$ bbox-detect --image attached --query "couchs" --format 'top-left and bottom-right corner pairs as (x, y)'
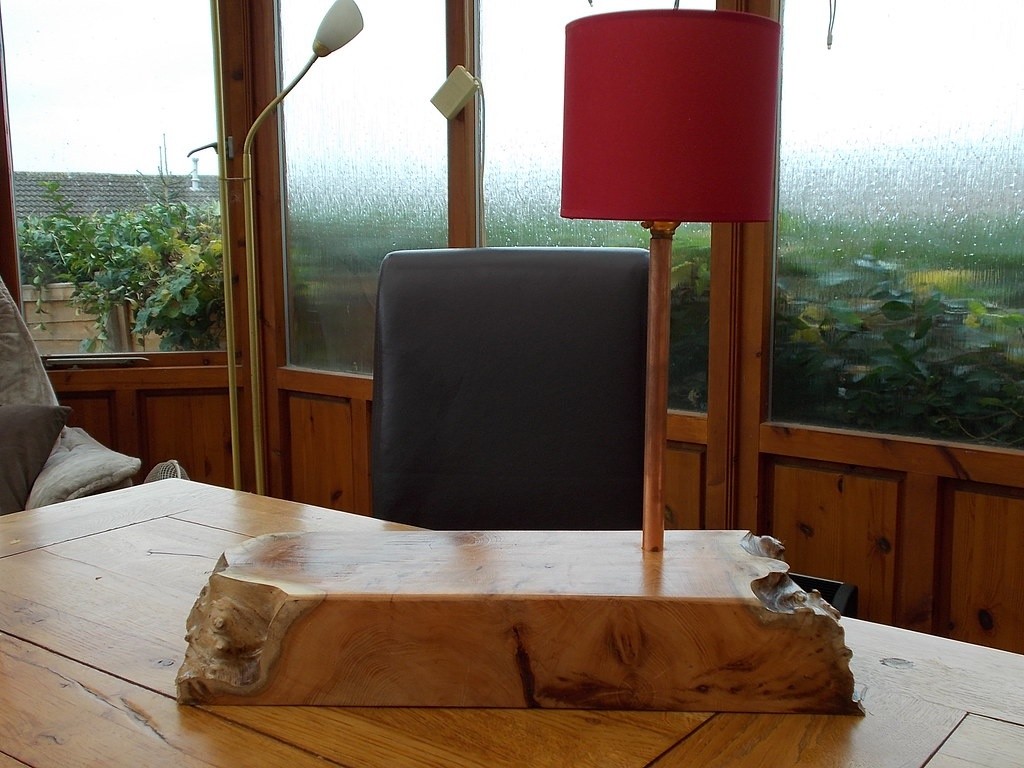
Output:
(0, 276), (142, 511)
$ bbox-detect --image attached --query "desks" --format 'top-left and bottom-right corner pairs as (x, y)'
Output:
(0, 475), (1024, 768)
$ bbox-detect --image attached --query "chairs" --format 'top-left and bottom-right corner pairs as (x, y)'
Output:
(367, 246), (647, 532)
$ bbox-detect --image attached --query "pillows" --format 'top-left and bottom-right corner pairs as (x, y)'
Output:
(0, 402), (73, 516)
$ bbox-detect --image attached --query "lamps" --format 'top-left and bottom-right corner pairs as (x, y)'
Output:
(174, 10), (866, 706)
(242, 0), (364, 497)
